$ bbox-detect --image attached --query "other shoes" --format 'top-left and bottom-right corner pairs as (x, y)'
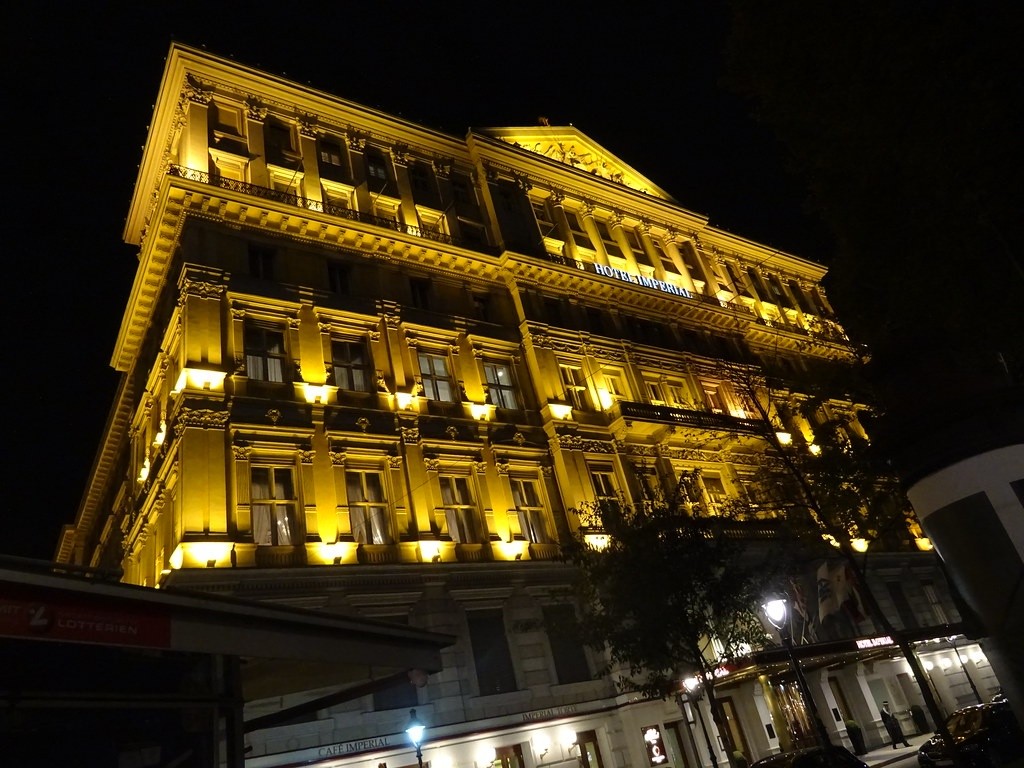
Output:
(906, 745), (913, 747)
(894, 747), (897, 749)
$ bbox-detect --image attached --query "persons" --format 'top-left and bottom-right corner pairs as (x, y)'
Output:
(795, 724), (804, 739)
(732, 751), (749, 768)
(880, 701), (913, 749)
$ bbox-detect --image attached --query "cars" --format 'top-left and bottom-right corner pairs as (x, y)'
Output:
(917, 690), (1012, 768)
(750, 744), (869, 768)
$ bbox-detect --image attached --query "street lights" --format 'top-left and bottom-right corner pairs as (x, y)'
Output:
(405, 707), (426, 768)
(761, 592), (834, 744)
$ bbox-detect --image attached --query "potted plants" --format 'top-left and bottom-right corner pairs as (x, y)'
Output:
(732, 750), (748, 768)
(846, 718), (867, 757)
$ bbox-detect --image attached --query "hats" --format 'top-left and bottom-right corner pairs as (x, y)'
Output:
(882, 701), (888, 705)
